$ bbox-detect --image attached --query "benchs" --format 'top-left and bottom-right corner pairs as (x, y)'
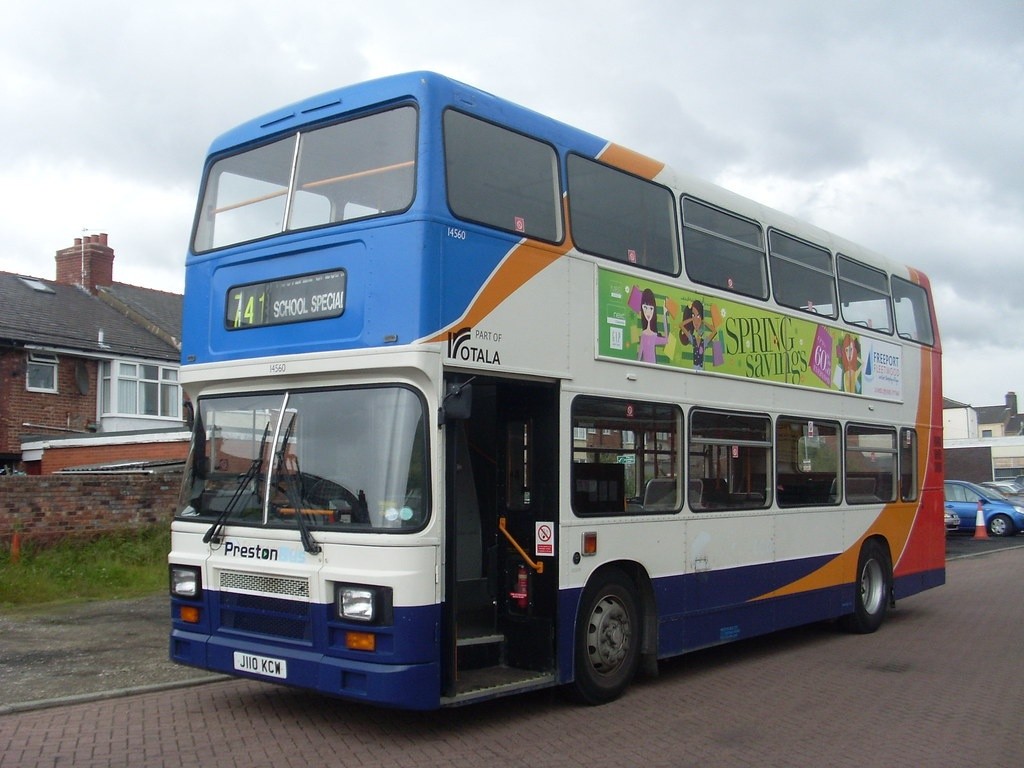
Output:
(625, 471), (913, 510)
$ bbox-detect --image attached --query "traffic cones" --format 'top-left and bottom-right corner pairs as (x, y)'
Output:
(8, 529), (22, 562)
(969, 499), (994, 542)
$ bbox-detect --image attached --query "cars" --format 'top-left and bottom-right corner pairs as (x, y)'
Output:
(978, 475), (1024, 508)
(943, 478), (1024, 538)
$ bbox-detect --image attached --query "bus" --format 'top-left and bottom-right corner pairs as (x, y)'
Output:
(165, 69), (948, 714)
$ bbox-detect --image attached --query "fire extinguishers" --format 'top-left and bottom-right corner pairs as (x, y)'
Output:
(518, 564), (528, 608)
